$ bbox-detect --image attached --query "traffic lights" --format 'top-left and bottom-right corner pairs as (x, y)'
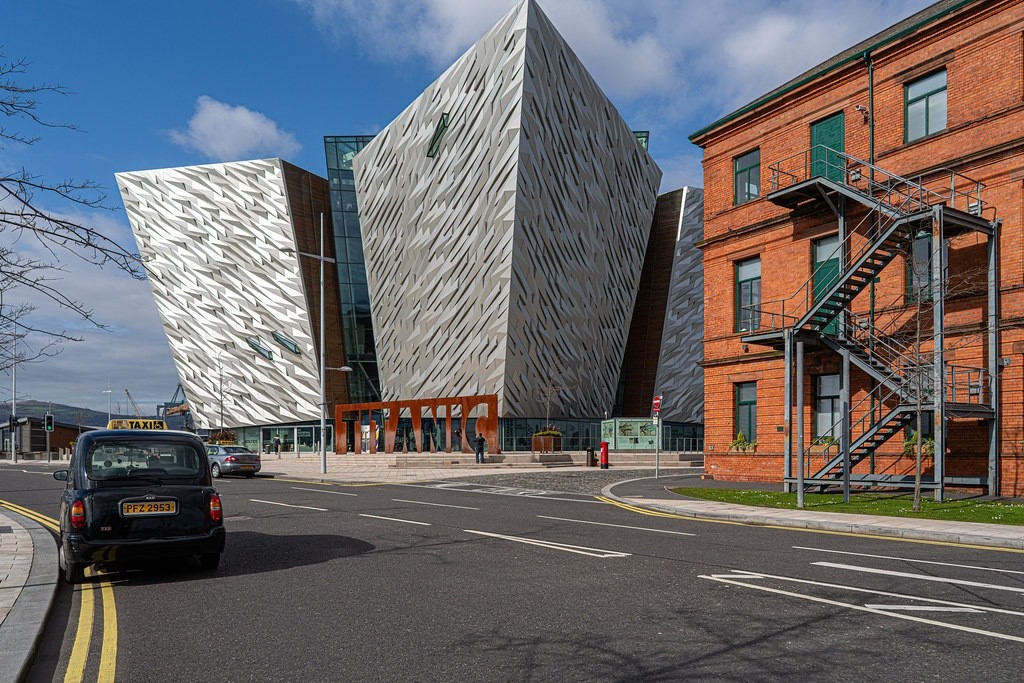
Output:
(44, 414), (54, 432)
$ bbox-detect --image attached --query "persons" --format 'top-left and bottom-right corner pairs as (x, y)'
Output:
(273, 434), (281, 455)
(473, 433), (486, 463)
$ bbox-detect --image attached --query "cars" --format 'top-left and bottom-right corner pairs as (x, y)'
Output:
(52, 419), (225, 585)
(205, 443), (262, 479)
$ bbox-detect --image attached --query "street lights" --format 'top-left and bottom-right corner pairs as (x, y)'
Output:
(280, 212), (353, 473)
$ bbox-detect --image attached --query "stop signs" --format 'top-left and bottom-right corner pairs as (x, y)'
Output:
(653, 396), (660, 407)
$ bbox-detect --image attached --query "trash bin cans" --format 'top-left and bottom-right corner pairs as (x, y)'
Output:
(265, 445), (271, 454)
(586, 446), (594, 467)
